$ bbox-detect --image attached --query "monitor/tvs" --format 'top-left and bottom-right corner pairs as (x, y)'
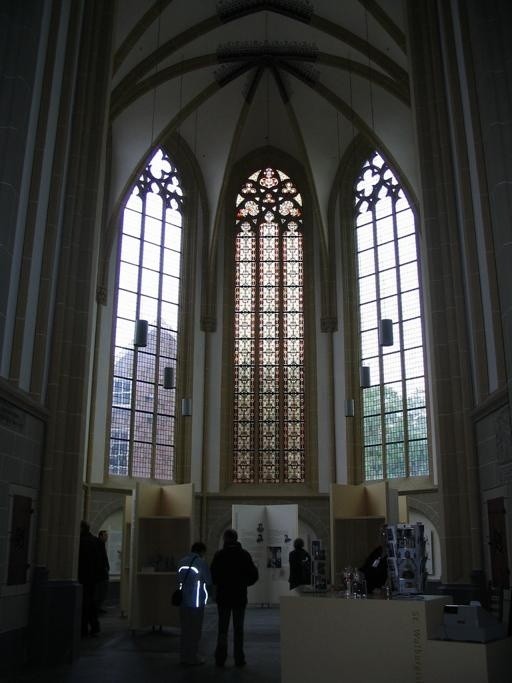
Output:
(433, 583), (488, 609)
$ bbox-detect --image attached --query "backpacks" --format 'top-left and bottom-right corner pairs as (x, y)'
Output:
(171, 589), (183, 607)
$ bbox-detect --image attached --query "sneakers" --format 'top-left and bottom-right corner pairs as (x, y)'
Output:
(179, 655), (207, 665)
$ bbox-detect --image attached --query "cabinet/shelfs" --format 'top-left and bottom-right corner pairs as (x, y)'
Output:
(329, 482), (389, 586)
(127, 481), (195, 636)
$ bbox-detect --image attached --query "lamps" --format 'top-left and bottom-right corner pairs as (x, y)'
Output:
(181, 399), (192, 416)
(345, 399), (354, 417)
(134, 320), (148, 347)
(164, 367), (174, 389)
(378, 319), (393, 346)
(360, 367), (370, 388)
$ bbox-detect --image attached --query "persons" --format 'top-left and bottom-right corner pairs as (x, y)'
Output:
(288, 538), (311, 590)
(177, 542), (212, 666)
(210, 529), (259, 667)
(95, 530), (110, 614)
(343, 541), (389, 595)
(80, 521), (107, 639)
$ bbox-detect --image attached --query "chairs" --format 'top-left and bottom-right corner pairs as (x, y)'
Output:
(485, 580), (504, 620)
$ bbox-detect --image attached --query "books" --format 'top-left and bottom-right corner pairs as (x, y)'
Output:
(383, 516), (430, 593)
